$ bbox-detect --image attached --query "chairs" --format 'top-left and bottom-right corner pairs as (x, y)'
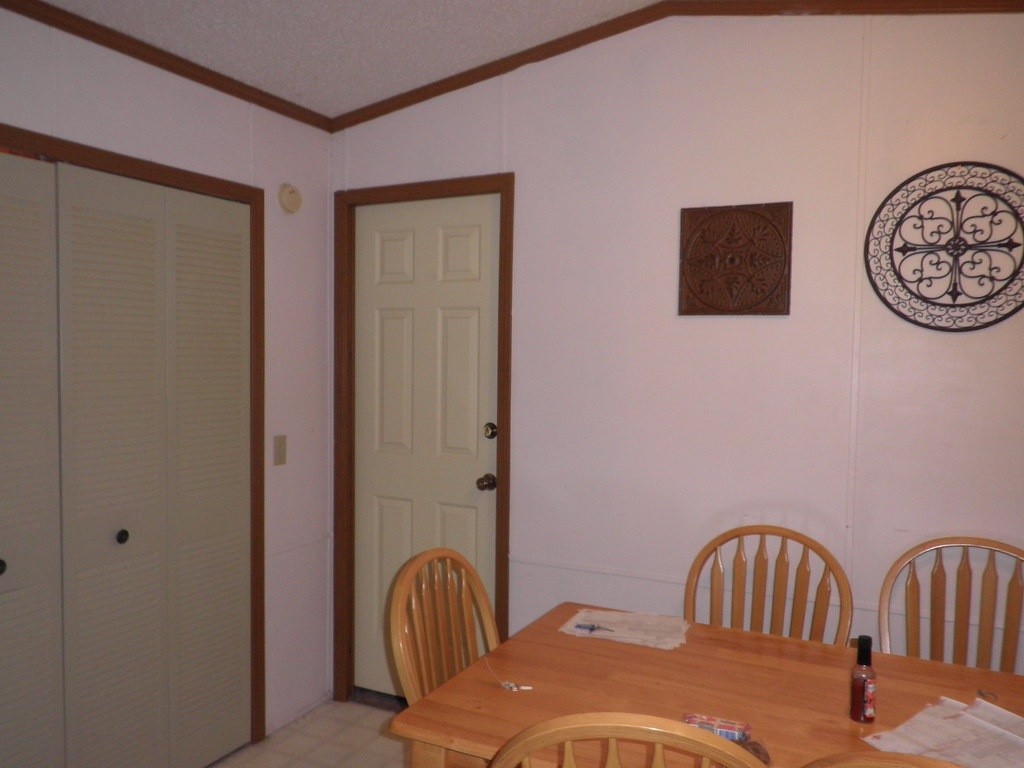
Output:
(878, 537), (1024, 674)
(488, 712), (767, 768)
(381, 548), (502, 709)
(684, 525), (852, 648)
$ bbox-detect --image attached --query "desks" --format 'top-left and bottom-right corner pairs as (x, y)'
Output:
(389, 602), (1024, 768)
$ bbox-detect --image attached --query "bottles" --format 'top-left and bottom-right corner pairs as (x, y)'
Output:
(850, 636), (878, 723)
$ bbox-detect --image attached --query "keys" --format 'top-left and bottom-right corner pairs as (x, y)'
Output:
(501, 681), (534, 692)
(575, 624), (615, 634)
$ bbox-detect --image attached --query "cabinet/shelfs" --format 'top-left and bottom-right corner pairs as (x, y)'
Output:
(0, 151), (251, 767)
